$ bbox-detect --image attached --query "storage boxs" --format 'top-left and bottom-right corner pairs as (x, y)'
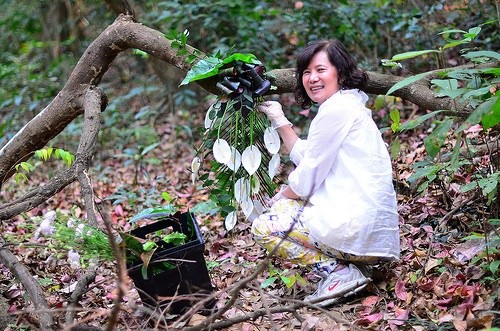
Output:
(125, 211), (215, 328)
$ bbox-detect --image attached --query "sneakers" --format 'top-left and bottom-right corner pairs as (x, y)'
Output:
(302, 263), (372, 309)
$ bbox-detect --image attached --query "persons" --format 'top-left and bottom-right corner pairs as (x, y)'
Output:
(251, 37), (400, 308)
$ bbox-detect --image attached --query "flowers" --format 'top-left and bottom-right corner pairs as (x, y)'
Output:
(178, 52), (284, 232)
